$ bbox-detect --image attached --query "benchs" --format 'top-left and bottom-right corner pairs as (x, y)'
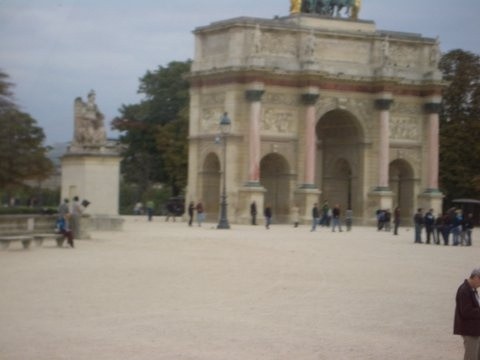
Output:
(34, 233), (63, 248)
(0, 236), (33, 250)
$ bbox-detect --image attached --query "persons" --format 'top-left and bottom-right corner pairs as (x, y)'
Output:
(310, 199), (353, 233)
(452, 268), (480, 360)
(250, 201), (258, 225)
(55, 195), (91, 247)
(133, 198), (144, 219)
(414, 207), (474, 246)
(291, 203), (300, 228)
(376, 205), (401, 235)
(263, 202), (272, 229)
(164, 200), (175, 222)
(187, 200), (204, 227)
(147, 200), (155, 220)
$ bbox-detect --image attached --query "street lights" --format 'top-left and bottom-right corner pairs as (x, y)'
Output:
(215, 112), (231, 228)
